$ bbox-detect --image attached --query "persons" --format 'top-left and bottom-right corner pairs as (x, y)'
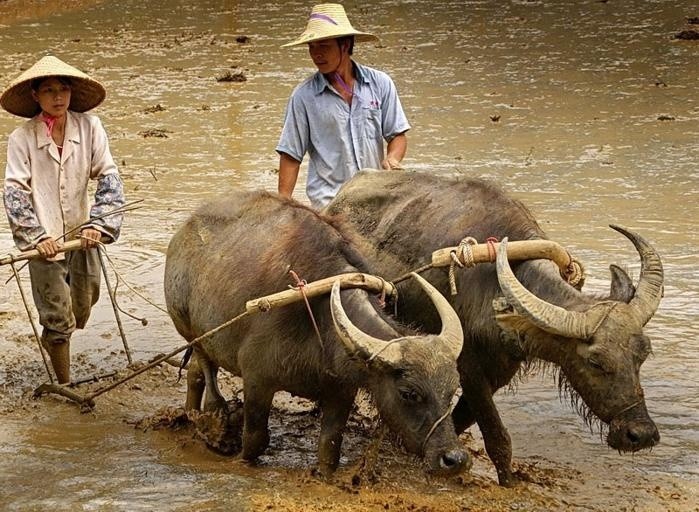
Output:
(275, 3), (412, 215)
(0, 54), (127, 386)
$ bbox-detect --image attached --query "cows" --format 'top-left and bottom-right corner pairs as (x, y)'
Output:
(316, 167), (665, 490)
(163, 189), (474, 481)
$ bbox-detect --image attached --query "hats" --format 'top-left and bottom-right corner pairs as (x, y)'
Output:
(0, 56), (106, 118)
(279, 3), (379, 50)
